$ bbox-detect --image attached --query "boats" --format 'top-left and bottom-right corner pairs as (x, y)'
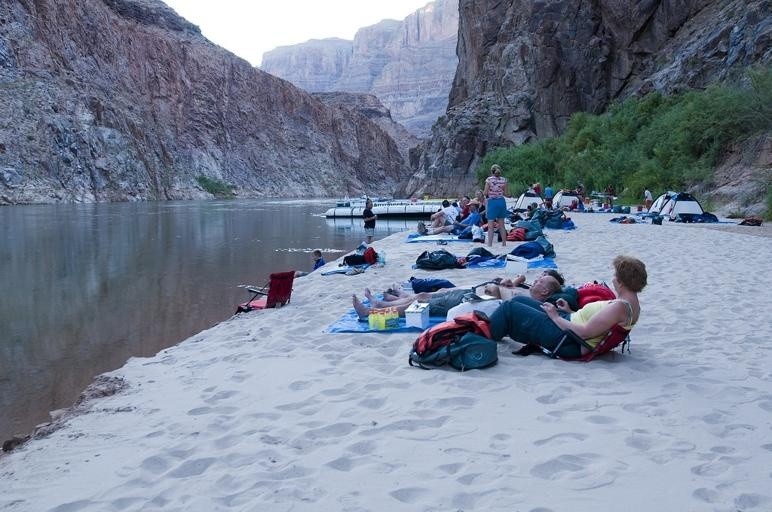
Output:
(325, 200), (454, 217)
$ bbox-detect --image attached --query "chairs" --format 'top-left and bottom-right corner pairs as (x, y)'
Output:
(526, 323), (632, 361)
(235, 271), (295, 314)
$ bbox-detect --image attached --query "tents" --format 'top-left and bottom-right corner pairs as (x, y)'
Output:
(648, 190), (704, 221)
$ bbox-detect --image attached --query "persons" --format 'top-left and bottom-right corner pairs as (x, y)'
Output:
(604, 184), (614, 207)
(351, 275), (561, 320)
(417, 164), (585, 247)
(383, 268), (565, 301)
(643, 187), (653, 210)
(293, 249), (326, 278)
(487, 255), (647, 359)
(362, 199), (378, 244)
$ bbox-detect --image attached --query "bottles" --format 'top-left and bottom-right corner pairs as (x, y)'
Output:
(368, 306), (398, 329)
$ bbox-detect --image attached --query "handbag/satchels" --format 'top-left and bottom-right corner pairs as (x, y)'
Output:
(344, 241), (378, 264)
(458, 225), (483, 241)
(409, 310), (498, 372)
